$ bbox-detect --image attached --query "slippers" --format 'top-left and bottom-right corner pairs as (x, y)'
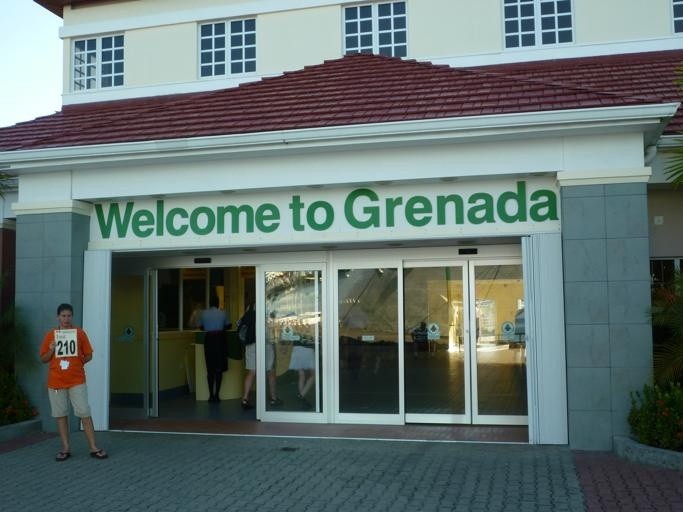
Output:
(91, 449), (107, 458)
(56, 452), (70, 461)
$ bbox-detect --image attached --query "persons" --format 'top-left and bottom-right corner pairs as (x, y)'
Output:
(289, 333), (315, 401)
(198, 296), (232, 404)
(187, 302), (201, 328)
(39, 303), (107, 461)
(236, 310), (284, 408)
(246, 296), (273, 340)
(411, 322), (428, 358)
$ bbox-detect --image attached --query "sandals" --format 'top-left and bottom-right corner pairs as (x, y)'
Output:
(270, 398), (283, 406)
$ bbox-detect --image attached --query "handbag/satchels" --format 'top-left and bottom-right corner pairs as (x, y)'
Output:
(236, 301), (255, 341)
